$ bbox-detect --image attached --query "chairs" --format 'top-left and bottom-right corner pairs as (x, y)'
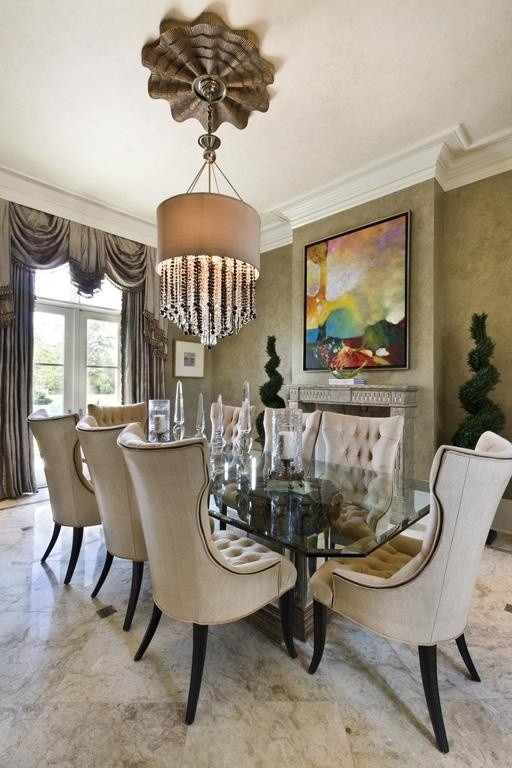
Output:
(306, 430), (512, 753)
(27, 410), (104, 584)
(88, 403), (150, 446)
(74, 415), (148, 634)
(209, 404), (406, 564)
(116, 422), (302, 726)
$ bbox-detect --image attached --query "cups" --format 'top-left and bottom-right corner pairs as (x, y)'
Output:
(149, 399), (170, 443)
(236, 457), (257, 493)
(272, 407), (304, 482)
(270, 492), (297, 542)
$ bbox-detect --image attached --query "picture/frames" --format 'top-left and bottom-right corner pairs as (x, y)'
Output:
(303, 211), (410, 372)
(174, 339), (206, 379)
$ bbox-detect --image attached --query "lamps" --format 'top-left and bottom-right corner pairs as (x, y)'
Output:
(142, 23), (273, 350)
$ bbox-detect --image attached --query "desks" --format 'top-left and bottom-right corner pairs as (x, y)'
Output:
(158, 439), (434, 646)
